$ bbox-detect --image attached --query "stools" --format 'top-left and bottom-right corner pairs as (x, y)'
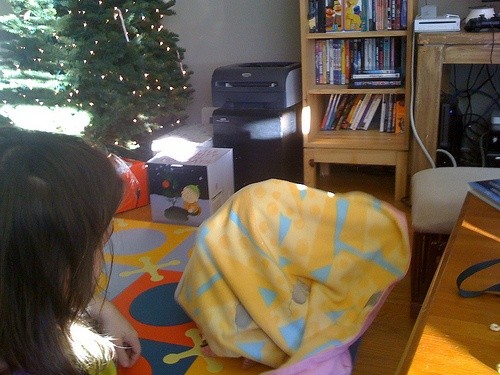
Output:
(411, 166), (499, 316)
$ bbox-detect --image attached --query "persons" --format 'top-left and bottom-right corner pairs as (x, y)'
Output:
(0, 127), (140, 375)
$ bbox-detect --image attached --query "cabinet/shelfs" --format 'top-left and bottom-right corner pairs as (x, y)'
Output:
(299, 0), (418, 200)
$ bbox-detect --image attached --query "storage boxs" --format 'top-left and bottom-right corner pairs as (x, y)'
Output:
(147, 148), (234, 226)
(113, 155), (150, 214)
(213, 103), (302, 192)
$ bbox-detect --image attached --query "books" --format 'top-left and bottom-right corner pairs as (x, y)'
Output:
(467, 178), (500, 211)
(308, 0), (407, 134)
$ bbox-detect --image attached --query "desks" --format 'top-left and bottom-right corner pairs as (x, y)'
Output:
(410, 32), (500, 176)
(395, 192), (500, 375)
